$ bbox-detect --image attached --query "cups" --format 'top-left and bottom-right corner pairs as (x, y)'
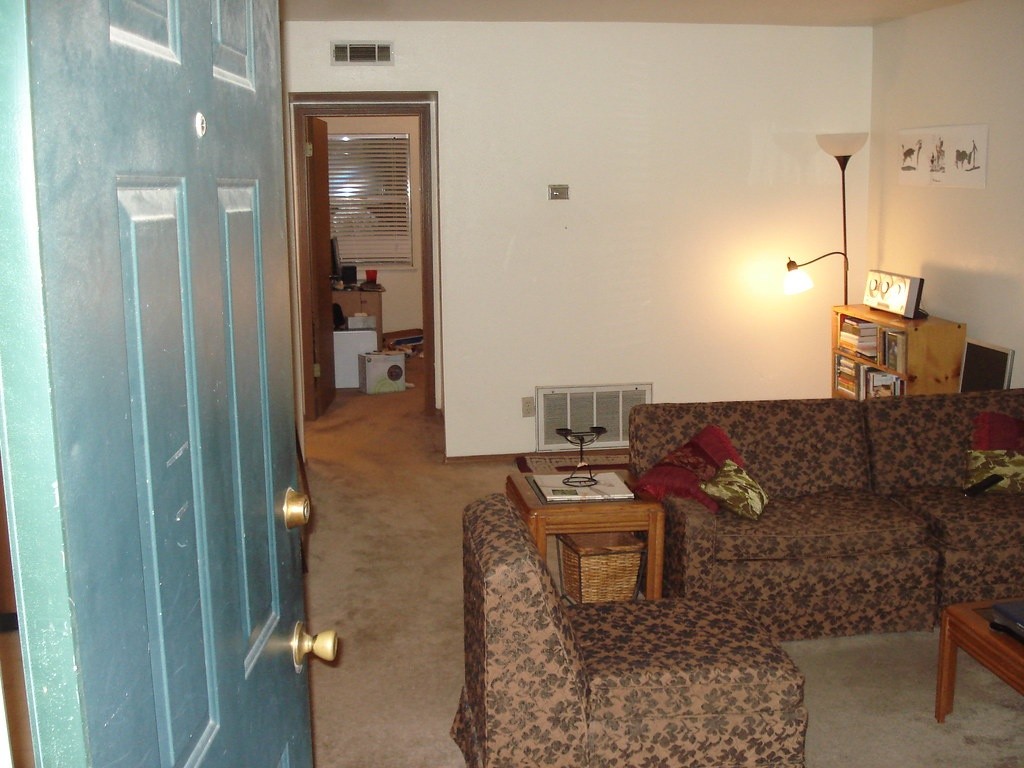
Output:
(365, 270), (377, 285)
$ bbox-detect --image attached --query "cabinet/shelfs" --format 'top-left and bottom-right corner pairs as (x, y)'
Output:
(332, 283), (386, 353)
(830, 304), (967, 401)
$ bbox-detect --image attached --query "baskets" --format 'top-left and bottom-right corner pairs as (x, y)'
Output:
(555, 531), (644, 604)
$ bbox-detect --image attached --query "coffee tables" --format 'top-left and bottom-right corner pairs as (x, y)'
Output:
(934, 597), (1024, 723)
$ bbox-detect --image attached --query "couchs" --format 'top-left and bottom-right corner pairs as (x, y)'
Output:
(628, 387), (1024, 643)
(451, 492), (809, 768)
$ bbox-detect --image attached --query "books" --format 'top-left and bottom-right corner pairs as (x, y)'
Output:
(837, 317), (905, 399)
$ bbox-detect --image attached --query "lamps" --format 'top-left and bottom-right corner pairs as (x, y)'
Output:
(782, 132), (870, 305)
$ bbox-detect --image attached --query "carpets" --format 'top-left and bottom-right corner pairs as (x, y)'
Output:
(516, 454), (630, 473)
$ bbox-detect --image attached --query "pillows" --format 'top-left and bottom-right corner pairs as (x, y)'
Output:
(698, 459), (770, 522)
(635, 422), (746, 514)
(971, 411), (1024, 452)
(962, 449), (1024, 494)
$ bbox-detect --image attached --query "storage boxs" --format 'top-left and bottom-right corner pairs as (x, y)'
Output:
(333, 331), (377, 389)
(357, 353), (406, 394)
(345, 316), (376, 330)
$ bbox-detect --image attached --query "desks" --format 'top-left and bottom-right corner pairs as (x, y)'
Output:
(502, 469), (667, 599)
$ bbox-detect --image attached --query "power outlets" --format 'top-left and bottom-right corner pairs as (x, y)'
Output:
(522, 397), (535, 417)
